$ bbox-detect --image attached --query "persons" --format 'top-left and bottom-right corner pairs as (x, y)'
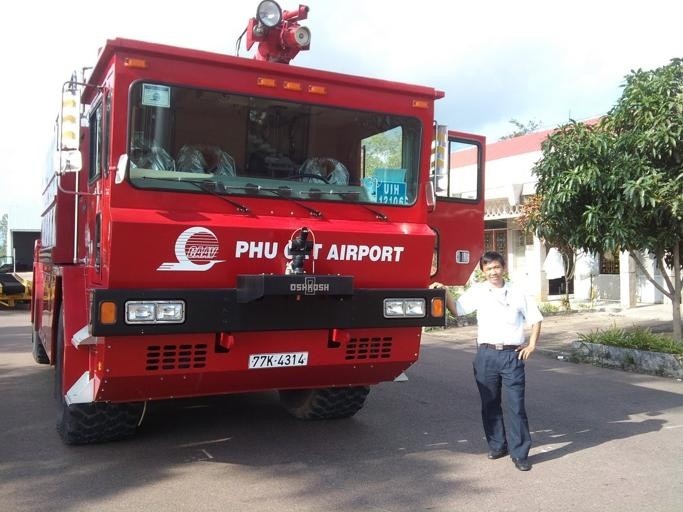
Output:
(427, 251), (544, 471)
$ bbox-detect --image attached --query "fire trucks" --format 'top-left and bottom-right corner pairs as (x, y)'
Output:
(31, 0), (486, 446)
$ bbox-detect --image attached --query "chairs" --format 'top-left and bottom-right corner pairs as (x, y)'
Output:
(141, 141), (348, 193)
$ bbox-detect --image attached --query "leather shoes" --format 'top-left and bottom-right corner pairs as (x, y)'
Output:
(512, 457), (531, 471)
(488, 447), (509, 460)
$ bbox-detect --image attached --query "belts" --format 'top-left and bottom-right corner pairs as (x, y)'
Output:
(479, 343), (522, 352)
